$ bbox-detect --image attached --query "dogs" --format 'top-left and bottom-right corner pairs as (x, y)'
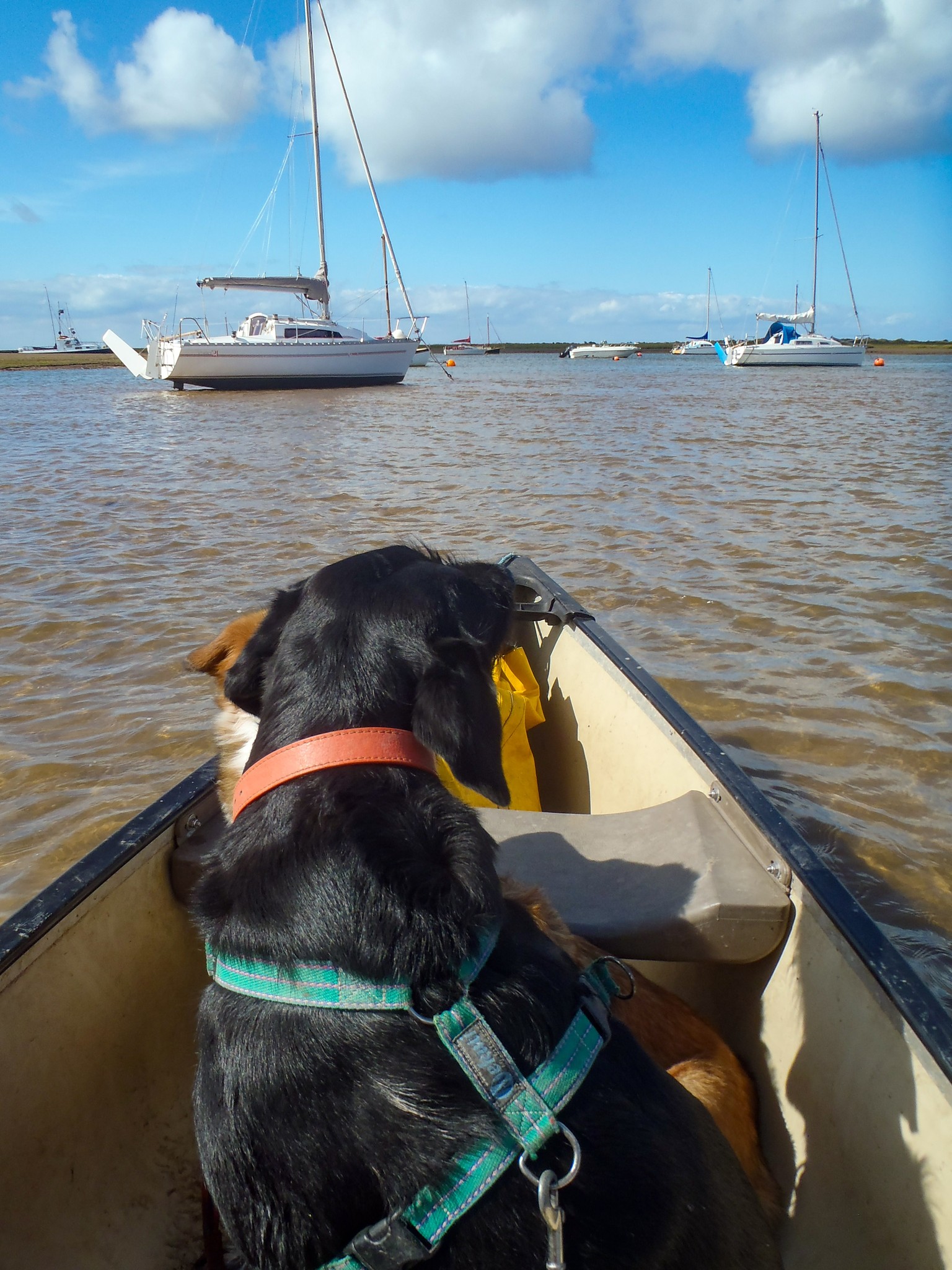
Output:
(165, 533), (791, 1270)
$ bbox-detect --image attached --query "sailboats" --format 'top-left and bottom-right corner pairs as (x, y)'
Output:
(18, 283), (114, 354)
(713, 108), (870, 368)
(669, 265), (719, 354)
(442, 280), (506, 356)
(101, 0), (430, 390)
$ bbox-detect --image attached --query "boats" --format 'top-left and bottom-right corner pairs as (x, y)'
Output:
(376, 233), (430, 371)
(558, 340), (643, 358)
